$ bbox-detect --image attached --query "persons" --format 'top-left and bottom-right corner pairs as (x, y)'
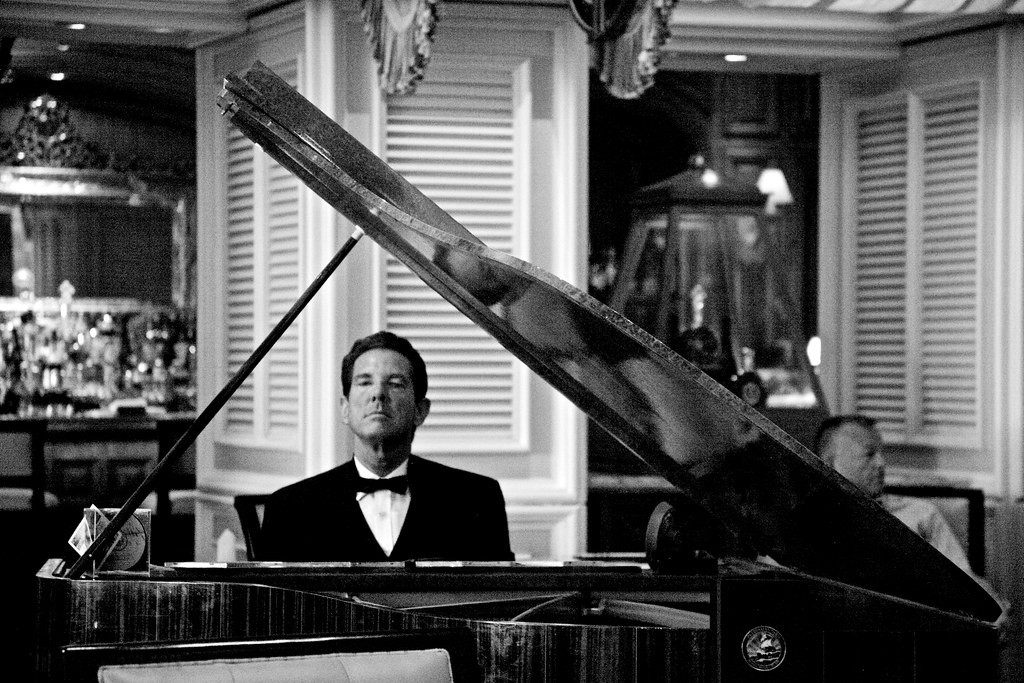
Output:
(815, 414), (975, 580)
(257, 330), (518, 562)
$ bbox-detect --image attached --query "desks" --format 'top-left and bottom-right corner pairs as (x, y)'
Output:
(0, 416), (197, 511)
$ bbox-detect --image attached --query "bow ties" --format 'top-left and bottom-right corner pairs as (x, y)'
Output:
(355, 474), (409, 495)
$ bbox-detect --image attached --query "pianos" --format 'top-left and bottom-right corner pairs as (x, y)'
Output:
(29, 59), (1014, 681)
